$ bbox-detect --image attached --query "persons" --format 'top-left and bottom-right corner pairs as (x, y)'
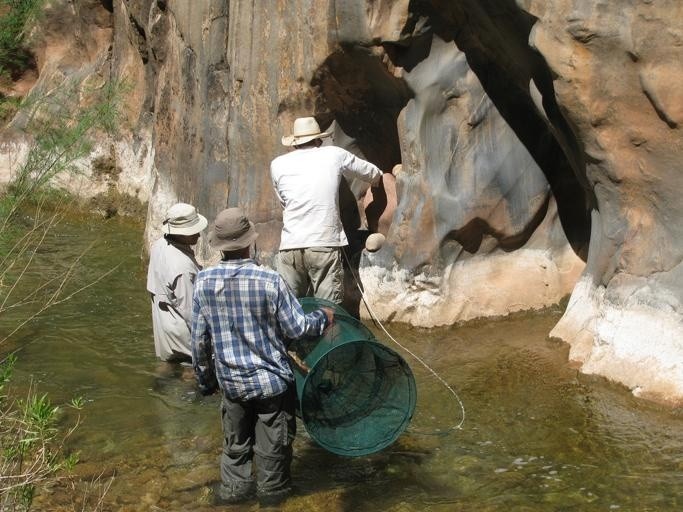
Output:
(270, 117), (383, 304)
(146, 203), (208, 367)
(191, 208), (334, 505)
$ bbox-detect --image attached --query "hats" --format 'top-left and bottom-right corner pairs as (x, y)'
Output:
(158, 203), (208, 237)
(208, 207), (260, 252)
(281, 116), (335, 145)
